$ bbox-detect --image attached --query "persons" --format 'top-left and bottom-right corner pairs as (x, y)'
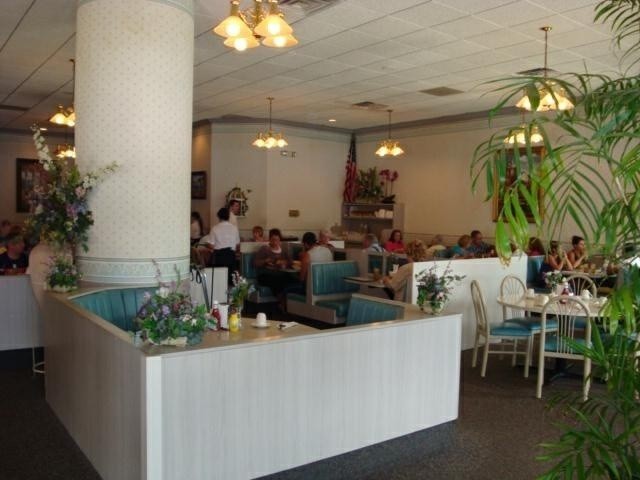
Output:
(192, 202), (637, 297)
(0, 219), (34, 275)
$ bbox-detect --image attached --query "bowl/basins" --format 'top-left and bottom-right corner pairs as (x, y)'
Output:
(378, 209), (394, 218)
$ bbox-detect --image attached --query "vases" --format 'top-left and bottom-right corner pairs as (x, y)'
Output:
(355, 196), (384, 204)
(381, 194), (395, 204)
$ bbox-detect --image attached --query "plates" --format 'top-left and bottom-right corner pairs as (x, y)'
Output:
(251, 323), (272, 328)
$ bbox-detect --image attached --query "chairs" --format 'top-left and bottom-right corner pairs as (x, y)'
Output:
(470, 273), (640, 402)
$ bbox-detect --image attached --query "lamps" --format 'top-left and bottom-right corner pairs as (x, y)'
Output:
(502, 88), (544, 145)
(55, 124), (75, 159)
(252, 98), (288, 148)
(515, 26), (576, 112)
(375, 109), (405, 157)
(211, 0), (298, 51)
(48, 59), (75, 127)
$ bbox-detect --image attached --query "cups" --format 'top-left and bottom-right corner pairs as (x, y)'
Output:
(583, 263), (596, 276)
(373, 267), (380, 283)
(255, 312), (267, 326)
(388, 271), (394, 282)
(525, 281), (608, 310)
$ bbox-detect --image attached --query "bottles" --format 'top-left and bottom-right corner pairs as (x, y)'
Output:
(212, 301), (221, 331)
(228, 308), (239, 334)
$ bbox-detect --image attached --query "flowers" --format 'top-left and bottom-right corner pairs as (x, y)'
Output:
(22, 121), (118, 291)
(378, 169), (398, 198)
(351, 165), (385, 204)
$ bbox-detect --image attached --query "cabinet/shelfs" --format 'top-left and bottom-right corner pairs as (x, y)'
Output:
(341, 201), (404, 240)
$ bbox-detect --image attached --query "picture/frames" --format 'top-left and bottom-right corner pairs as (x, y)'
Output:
(16, 158), (57, 214)
(492, 145), (546, 223)
(191, 169), (209, 202)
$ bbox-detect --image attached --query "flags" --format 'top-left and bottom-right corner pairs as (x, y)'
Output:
(343, 135), (361, 203)
